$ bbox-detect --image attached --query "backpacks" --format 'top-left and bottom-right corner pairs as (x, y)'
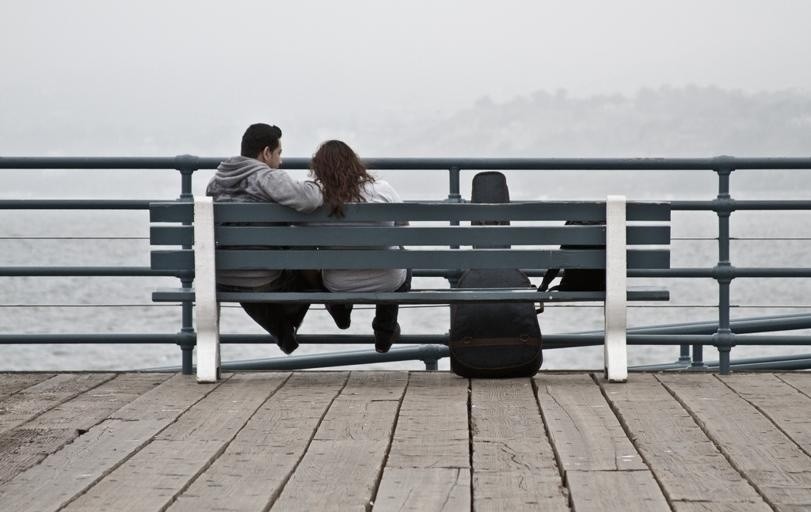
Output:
(560, 220), (606, 292)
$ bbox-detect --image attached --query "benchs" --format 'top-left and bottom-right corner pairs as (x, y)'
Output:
(149, 196), (673, 384)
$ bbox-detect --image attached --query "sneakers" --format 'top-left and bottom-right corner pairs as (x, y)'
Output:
(325, 303), (350, 329)
(374, 322), (400, 353)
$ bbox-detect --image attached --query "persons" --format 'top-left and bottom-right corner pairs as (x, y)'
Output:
(310, 138), (412, 353)
(202, 122), (325, 356)
